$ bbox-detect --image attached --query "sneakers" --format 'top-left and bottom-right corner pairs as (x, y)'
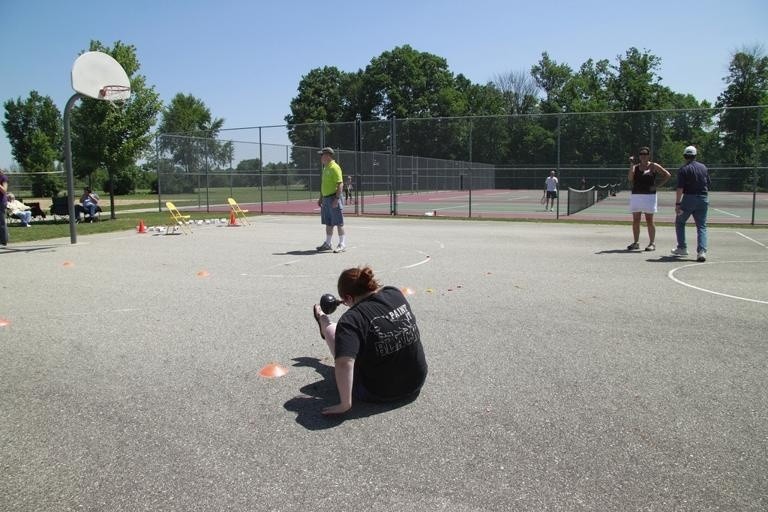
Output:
(697, 250), (707, 261)
(645, 243), (656, 251)
(313, 303), (330, 339)
(670, 246), (689, 256)
(315, 241), (333, 251)
(545, 206), (554, 213)
(23, 222), (31, 227)
(0, 243), (8, 249)
(75, 219), (95, 224)
(334, 244), (347, 253)
(627, 242), (640, 249)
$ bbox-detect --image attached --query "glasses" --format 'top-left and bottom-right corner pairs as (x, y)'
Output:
(639, 152), (648, 156)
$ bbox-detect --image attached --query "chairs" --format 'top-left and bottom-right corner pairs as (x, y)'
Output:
(228, 198), (250, 227)
(7, 196), (100, 225)
(165, 201), (191, 234)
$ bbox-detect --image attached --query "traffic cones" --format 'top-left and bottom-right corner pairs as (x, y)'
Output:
(138, 218), (147, 233)
(229, 208), (238, 226)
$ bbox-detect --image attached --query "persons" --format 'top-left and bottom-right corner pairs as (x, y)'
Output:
(316, 147), (346, 253)
(628, 146), (671, 251)
(314, 266), (427, 415)
(6, 194), (32, 227)
(74, 187), (98, 225)
(671, 146), (711, 261)
(345, 176), (354, 205)
(0, 169), (8, 248)
(544, 170), (558, 211)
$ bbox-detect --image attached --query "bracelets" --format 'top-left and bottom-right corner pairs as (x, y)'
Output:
(336, 197), (339, 199)
(675, 203), (680, 205)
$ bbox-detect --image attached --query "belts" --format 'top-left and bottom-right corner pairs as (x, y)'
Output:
(323, 193), (336, 199)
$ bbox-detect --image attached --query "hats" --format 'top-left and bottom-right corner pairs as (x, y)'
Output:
(316, 146), (334, 156)
(682, 145), (698, 158)
